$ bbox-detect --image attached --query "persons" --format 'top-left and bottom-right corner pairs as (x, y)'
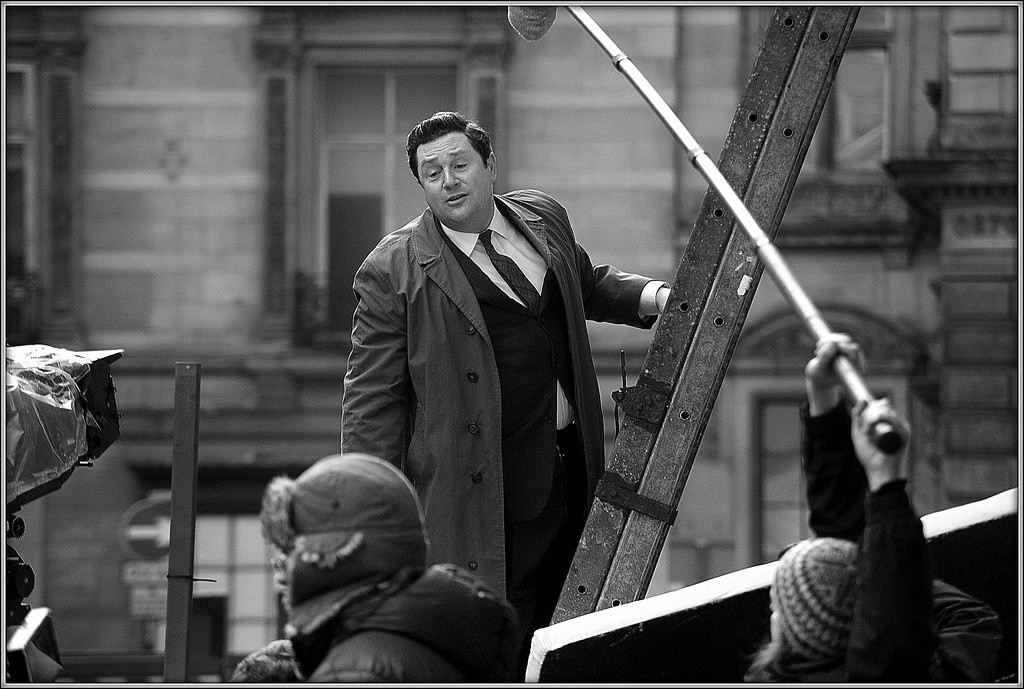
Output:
(232, 452), (522, 683)
(743, 332), (1003, 684)
(341, 111), (672, 637)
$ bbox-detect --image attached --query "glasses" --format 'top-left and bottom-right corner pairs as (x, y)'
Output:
(270, 554), (289, 573)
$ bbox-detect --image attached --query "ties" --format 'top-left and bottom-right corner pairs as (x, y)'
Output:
(479, 229), (541, 315)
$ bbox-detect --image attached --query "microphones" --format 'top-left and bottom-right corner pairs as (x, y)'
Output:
(507, 6), (558, 41)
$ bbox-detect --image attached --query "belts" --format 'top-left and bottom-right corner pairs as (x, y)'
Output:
(557, 421), (585, 461)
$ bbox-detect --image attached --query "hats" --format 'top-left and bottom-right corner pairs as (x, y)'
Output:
(769, 537), (862, 662)
(259, 453), (426, 628)
